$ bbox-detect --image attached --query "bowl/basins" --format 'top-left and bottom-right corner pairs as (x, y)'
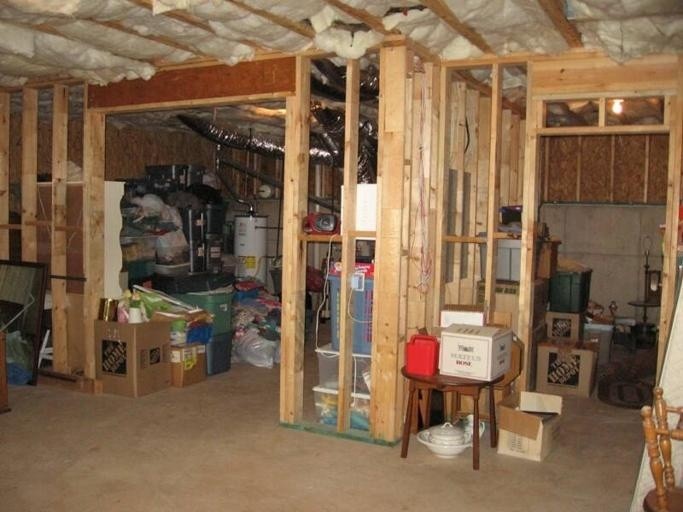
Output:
(417, 427), (474, 459)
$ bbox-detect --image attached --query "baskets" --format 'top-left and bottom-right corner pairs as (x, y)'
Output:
(324, 274), (374, 356)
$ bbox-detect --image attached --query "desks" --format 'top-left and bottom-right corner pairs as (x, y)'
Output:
(400, 364), (506, 470)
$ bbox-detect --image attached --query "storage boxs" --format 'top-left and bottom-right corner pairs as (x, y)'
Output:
(436, 232), (616, 464)
(310, 272), (375, 432)
(93, 285), (236, 399)
